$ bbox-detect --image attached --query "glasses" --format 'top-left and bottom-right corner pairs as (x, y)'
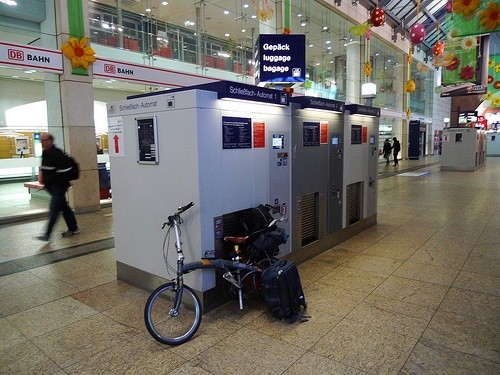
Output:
(40, 139), (48, 142)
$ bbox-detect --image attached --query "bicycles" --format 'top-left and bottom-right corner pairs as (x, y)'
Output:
(144, 202), (281, 346)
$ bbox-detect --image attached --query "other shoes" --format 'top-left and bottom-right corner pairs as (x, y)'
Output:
(62, 227), (80, 237)
(393, 165), (396, 166)
(37, 235), (50, 242)
(386, 164), (389, 166)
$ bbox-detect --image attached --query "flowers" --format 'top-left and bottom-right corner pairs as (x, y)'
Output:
(61, 38), (96, 69)
(492, 97), (500, 106)
(461, 35), (478, 50)
(365, 62), (373, 77)
(484, 90), (493, 100)
(453, 0), (481, 17)
(494, 80), (500, 89)
(445, 57), (460, 70)
(406, 80), (416, 92)
(489, 59), (495, 68)
(407, 108), (412, 119)
(494, 63), (500, 72)
(480, 1), (500, 31)
(488, 75), (494, 83)
(460, 64), (474, 80)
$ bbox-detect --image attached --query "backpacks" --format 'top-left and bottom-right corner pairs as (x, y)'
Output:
(63, 152), (81, 181)
(229, 204), (290, 301)
(259, 259), (311, 324)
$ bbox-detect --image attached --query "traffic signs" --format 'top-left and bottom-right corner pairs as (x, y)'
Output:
(107, 117), (125, 157)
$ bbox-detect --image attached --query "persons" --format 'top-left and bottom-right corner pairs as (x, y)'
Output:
(390, 137), (400, 167)
(33, 134), (80, 242)
(19, 144), (25, 158)
(382, 138), (392, 166)
